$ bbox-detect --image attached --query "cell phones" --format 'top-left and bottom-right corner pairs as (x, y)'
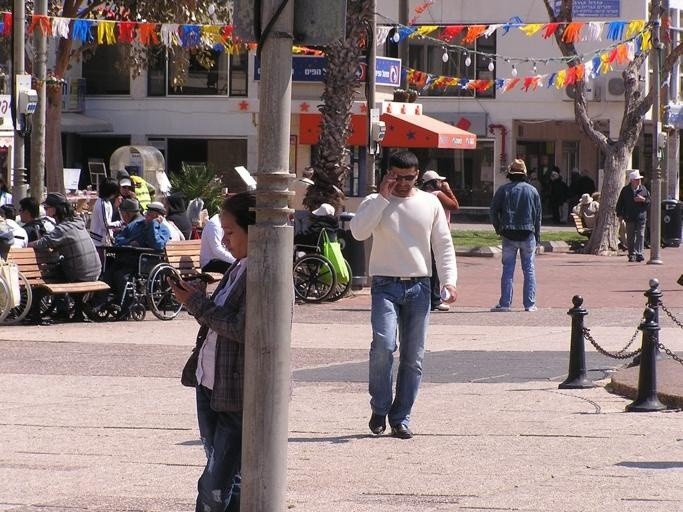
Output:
(169, 274), (184, 290)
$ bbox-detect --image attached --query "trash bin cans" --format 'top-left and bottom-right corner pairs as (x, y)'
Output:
(661, 198), (683, 247)
(337, 212), (372, 289)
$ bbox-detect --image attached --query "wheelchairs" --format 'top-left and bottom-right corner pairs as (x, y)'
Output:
(18, 219), (86, 319)
(0, 232), (30, 325)
(292, 225), (355, 306)
(83, 239), (190, 323)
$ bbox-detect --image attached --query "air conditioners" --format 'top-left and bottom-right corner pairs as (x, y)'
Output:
(560, 72), (596, 103)
(604, 70), (629, 101)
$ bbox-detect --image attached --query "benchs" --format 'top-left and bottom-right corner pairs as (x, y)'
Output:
(163, 235), (225, 301)
(570, 210), (595, 240)
(0, 241), (114, 327)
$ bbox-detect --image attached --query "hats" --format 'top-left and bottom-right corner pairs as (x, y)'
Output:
(312, 203), (335, 216)
(145, 202), (167, 215)
(629, 172), (643, 180)
(422, 171), (447, 183)
(579, 194), (593, 204)
(40, 192), (66, 205)
(507, 158), (527, 175)
(118, 199), (141, 212)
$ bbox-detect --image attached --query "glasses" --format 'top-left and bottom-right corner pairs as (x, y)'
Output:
(393, 173), (414, 181)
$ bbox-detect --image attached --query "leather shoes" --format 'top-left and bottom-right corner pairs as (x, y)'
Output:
(369, 412), (386, 434)
(391, 423), (412, 438)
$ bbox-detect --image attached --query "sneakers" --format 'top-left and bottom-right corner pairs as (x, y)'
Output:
(491, 303), (510, 312)
(525, 304), (538, 312)
(431, 303), (449, 310)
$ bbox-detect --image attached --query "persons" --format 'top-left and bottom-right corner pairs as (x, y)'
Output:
(617, 169), (651, 263)
(420, 168), (459, 312)
(296, 203), (339, 260)
(167, 193), (256, 511)
(489, 158), (542, 311)
(299, 167), (314, 184)
(199, 213), (238, 274)
(1, 177), (183, 320)
(529, 164), (601, 241)
(349, 150), (458, 439)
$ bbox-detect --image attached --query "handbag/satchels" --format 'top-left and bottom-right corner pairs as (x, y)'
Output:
(181, 348), (198, 387)
(0, 261), (20, 309)
(320, 231), (350, 283)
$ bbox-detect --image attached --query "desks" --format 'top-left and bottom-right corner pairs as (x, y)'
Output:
(66, 191), (100, 217)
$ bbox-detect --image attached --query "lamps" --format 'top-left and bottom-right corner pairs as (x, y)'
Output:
(389, 23), (520, 79)
